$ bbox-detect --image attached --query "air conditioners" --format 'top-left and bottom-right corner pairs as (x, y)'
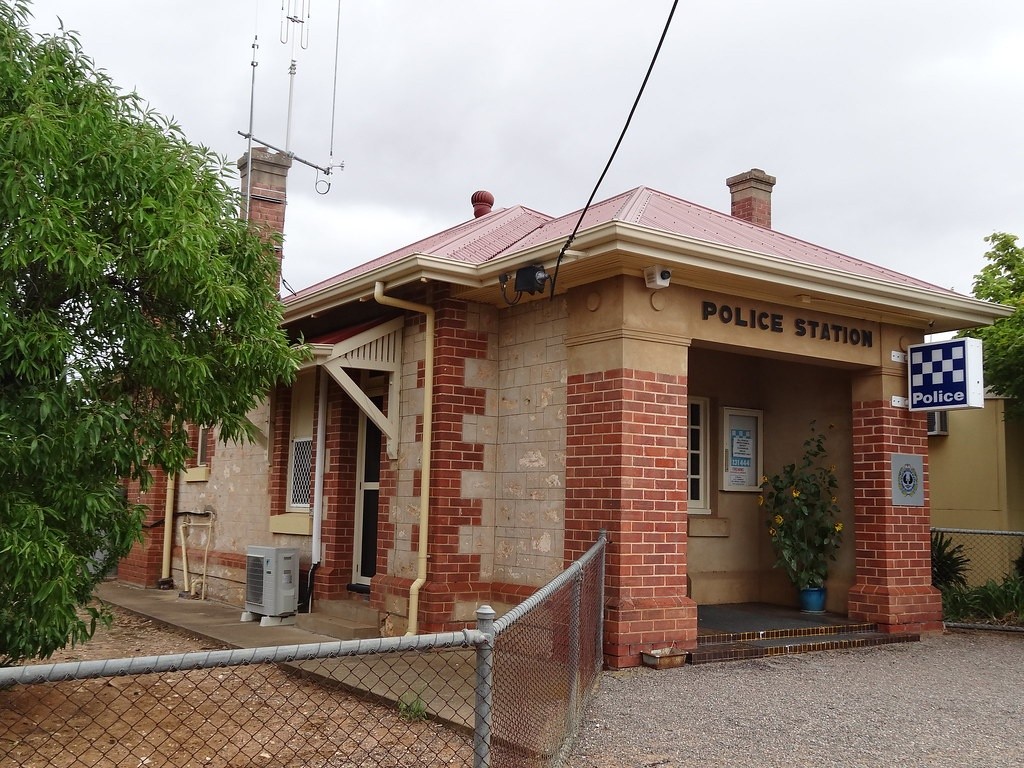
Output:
(927, 412), (949, 436)
(244, 545), (298, 618)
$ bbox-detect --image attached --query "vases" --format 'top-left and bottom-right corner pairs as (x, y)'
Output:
(797, 589), (827, 616)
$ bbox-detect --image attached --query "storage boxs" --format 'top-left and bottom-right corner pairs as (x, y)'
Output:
(641, 647), (689, 671)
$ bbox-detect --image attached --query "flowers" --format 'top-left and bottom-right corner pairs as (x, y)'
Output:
(756, 418), (844, 590)
